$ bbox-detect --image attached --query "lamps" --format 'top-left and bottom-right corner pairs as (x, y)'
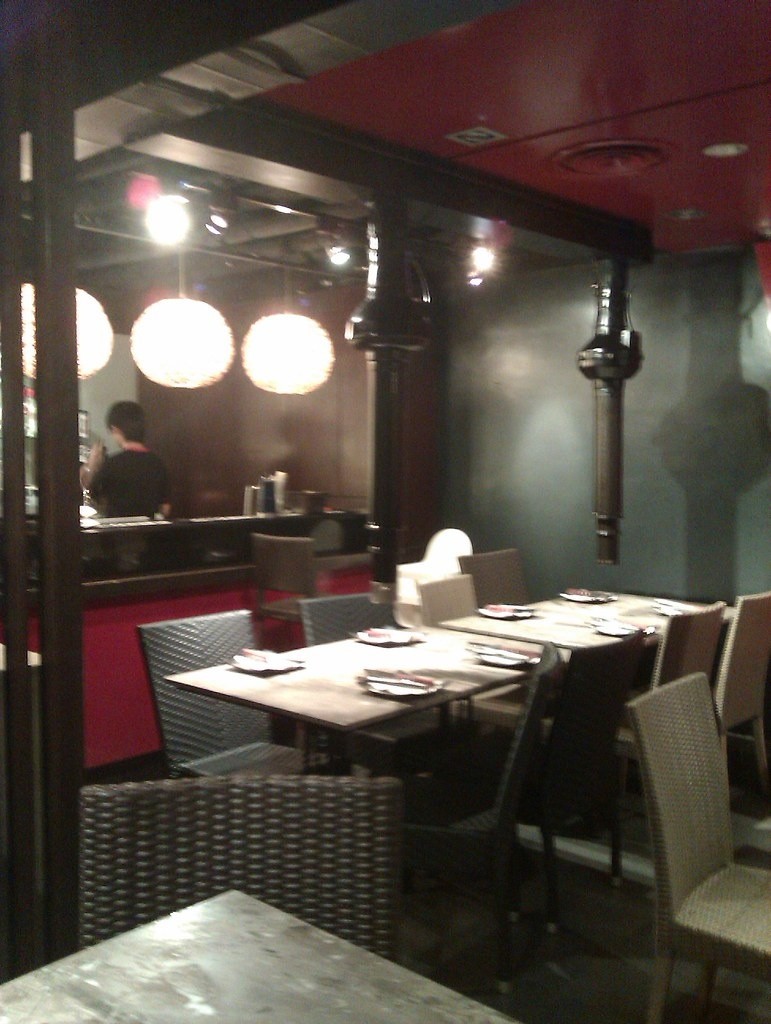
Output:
(239, 238), (337, 396)
(204, 186), (241, 237)
(130, 248), (237, 390)
(21, 281), (114, 379)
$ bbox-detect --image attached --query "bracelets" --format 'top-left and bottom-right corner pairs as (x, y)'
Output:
(84, 466), (97, 476)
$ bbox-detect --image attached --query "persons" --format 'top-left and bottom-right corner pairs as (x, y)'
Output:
(80, 401), (172, 521)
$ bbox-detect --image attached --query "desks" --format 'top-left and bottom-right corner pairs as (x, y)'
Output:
(0, 890), (524, 1024)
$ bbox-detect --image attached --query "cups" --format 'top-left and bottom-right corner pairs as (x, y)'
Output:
(259, 479), (275, 514)
(243, 486), (259, 516)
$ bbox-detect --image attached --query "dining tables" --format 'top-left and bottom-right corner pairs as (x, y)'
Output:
(164, 592), (734, 891)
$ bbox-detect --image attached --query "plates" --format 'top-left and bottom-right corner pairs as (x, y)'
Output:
(351, 630), (422, 644)
(473, 647), (541, 664)
(652, 605), (689, 616)
(591, 623), (639, 635)
(355, 677), (448, 695)
(226, 658), (304, 672)
(475, 607), (534, 618)
(560, 592), (615, 602)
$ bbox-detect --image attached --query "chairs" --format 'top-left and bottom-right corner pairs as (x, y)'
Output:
(451, 546), (553, 740)
(248, 530), (332, 645)
(300, 588), (474, 747)
(434, 631), (625, 888)
(712, 589), (771, 795)
(140, 608), (331, 781)
(392, 654), (564, 982)
(77, 773), (400, 957)
(603, 600), (726, 759)
(623, 672), (771, 1024)
